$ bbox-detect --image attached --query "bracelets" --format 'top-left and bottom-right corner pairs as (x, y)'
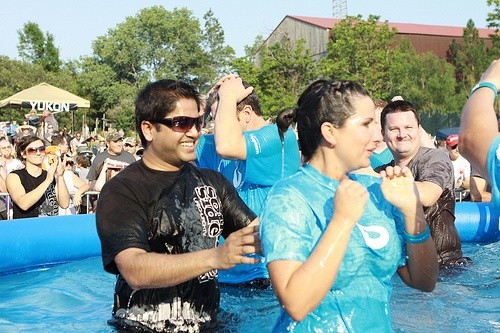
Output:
(469, 81), (497, 104)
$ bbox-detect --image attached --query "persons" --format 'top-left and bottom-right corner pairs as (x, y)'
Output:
(253, 77), (440, 333)
(369, 95), (470, 273)
(94, 80), (264, 333)
(459, 57), (499, 201)
(1, 120), (143, 222)
(192, 72), (302, 289)
(434, 134), (492, 202)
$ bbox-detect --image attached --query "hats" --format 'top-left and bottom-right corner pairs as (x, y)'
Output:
(445, 133), (459, 147)
(105, 131), (123, 142)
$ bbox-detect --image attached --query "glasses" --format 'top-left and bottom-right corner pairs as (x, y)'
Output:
(145, 115), (205, 133)
(447, 144), (458, 149)
(23, 146), (46, 155)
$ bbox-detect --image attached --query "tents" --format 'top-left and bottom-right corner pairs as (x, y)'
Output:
(0, 80), (90, 139)
